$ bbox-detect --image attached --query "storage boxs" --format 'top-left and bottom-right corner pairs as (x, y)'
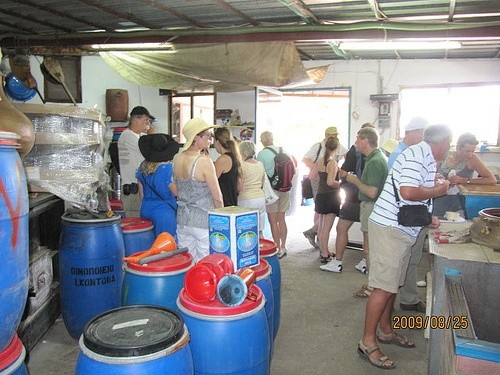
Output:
(18, 249), (62, 353)
(208, 204), (259, 272)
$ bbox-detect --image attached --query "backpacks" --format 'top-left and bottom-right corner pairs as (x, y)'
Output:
(262, 146), (295, 192)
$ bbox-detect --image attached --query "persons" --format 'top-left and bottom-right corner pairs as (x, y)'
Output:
(434, 132), (497, 219)
(257, 131), (298, 258)
(358, 125), (452, 368)
(302, 123), (375, 273)
(118, 106), (266, 263)
(346, 127), (388, 297)
(388, 118), (427, 312)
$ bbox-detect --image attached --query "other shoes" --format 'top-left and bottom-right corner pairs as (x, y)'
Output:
(278, 248), (287, 259)
(400, 301), (426, 313)
(303, 229), (319, 249)
(416, 280), (426, 287)
(352, 286), (372, 298)
(320, 252), (336, 262)
(355, 259), (367, 274)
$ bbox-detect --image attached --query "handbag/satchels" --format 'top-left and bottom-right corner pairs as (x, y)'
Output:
(302, 178), (313, 199)
(397, 204), (432, 226)
(262, 173), (278, 205)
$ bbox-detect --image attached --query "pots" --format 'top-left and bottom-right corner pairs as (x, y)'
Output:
(478, 207), (500, 242)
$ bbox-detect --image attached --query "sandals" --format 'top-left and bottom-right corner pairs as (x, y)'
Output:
(357, 341), (395, 369)
(377, 331), (416, 348)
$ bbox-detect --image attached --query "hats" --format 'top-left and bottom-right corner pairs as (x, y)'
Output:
(130, 106), (155, 119)
(406, 117), (428, 131)
(139, 133), (179, 159)
(182, 118), (220, 152)
(325, 127), (339, 135)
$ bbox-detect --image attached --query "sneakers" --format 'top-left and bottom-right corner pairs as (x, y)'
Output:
(320, 260), (341, 273)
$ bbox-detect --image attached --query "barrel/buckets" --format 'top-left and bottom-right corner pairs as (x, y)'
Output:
(176, 284), (270, 375)
(302, 198), (313, 206)
(109, 200), (125, 218)
(113, 128), (129, 141)
(121, 249), (196, 310)
(0, 132), (29, 354)
(249, 259), (274, 362)
(259, 238), (281, 340)
(120, 217), (155, 258)
(75, 304), (194, 375)
(58, 210), (125, 339)
(0, 334), (29, 375)
